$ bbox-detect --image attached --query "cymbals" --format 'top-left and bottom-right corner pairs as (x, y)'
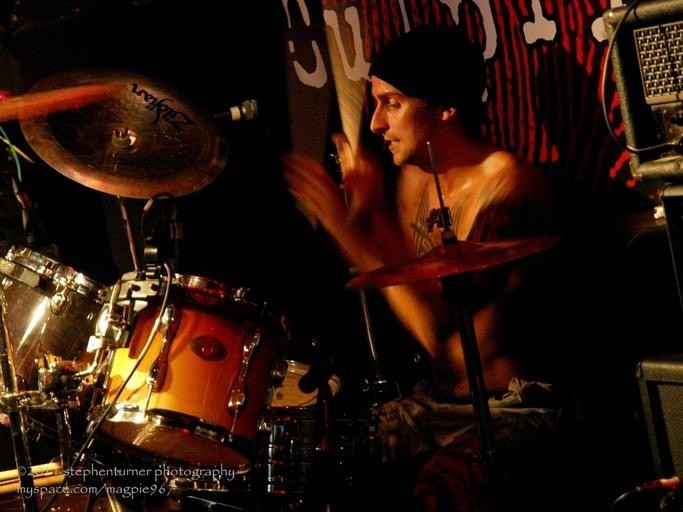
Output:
(20, 73), (231, 200)
(345, 238), (558, 289)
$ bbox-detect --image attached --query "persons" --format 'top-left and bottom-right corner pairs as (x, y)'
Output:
(276, 21), (575, 507)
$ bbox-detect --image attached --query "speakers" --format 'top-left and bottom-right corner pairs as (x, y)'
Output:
(635, 350), (683, 498)
(599, 1), (683, 182)
(661, 185), (683, 307)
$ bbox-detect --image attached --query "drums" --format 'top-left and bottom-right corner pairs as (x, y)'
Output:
(270, 358), (340, 410)
(271, 417), (370, 511)
(0, 245), (104, 451)
(89, 269), (269, 470)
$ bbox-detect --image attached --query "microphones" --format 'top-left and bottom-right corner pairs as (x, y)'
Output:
(11, 176), (36, 244)
(214, 97), (260, 122)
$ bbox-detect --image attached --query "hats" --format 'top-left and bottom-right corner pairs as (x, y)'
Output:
(368, 21), (486, 122)
